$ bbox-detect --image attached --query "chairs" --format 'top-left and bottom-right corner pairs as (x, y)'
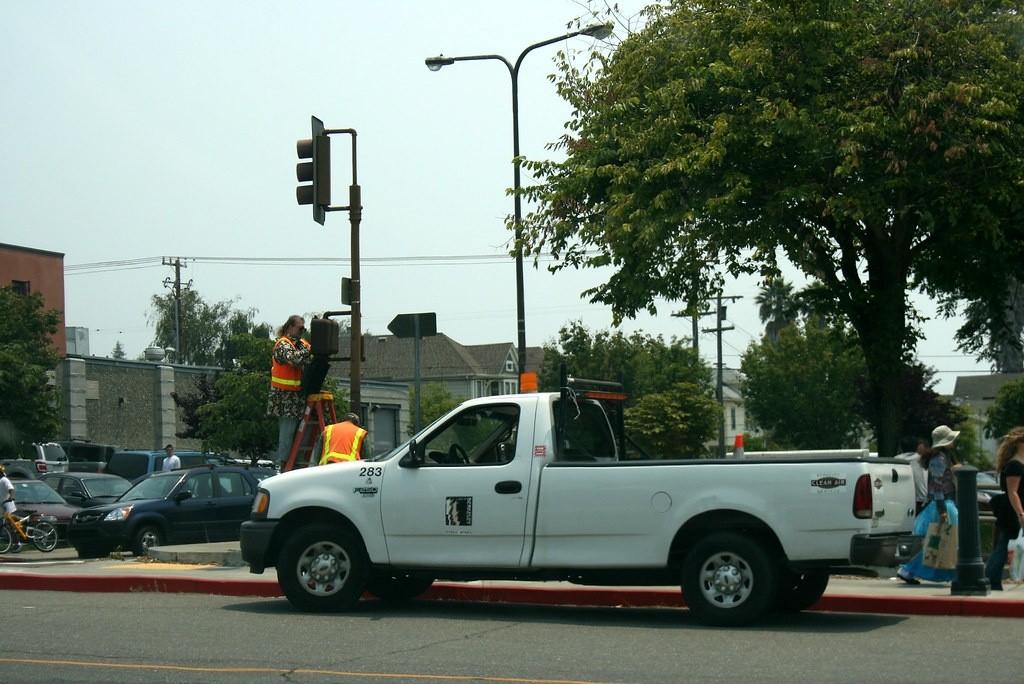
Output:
(496, 442), (512, 462)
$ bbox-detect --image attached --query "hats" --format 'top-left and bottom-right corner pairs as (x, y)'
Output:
(931, 425), (960, 449)
(899, 435), (920, 453)
(162, 444), (173, 450)
(345, 412), (360, 425)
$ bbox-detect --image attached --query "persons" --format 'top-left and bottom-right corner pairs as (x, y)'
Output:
(266, 316), (314, 476)
(308, 412), (375, 467)
(986, 426), (1024, 590)
(0, 465), (22, 552)
(895, 425), (960, 584)
(162, 444), (180, 470)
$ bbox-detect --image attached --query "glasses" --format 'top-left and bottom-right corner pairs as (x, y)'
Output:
(292, 323), (307, 333)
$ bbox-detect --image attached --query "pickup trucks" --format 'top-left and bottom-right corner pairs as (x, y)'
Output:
(239, 381), (928, 627)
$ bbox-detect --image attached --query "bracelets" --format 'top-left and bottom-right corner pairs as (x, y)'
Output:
(1018, 513), (1024, 517)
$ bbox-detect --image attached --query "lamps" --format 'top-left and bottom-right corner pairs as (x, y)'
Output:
(120, 397), (128, 404)
(371, 404), (381, 415)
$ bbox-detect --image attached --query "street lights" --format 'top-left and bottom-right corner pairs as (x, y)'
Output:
(423, 20), (613, 391)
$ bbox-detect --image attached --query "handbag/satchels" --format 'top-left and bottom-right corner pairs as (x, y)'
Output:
(1007, 526), (1024, 583)
(923, 516), (958, 568)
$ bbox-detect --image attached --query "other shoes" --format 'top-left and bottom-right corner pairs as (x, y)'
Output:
(896, 567), (920, 584)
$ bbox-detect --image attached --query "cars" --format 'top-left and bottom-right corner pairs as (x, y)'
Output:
(10, 479), (83, 538)
(976, 470), (1005, 507)
(32, 470), (145, 508)
(236, 458), (280, 481)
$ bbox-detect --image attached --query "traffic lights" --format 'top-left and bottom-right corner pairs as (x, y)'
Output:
(296, 114), (332, 226)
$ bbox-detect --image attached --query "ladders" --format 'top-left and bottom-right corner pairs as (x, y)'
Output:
(280, 392), (338, 471)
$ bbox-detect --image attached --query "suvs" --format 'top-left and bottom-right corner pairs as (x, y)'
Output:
(66, 462), (276, 560)
(0, 442), (70, 480)
(104, 446), (240, 494)
(58, 440), (119, 470)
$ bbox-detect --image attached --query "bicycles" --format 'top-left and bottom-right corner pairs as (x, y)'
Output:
(0, 497), (58, 554)
